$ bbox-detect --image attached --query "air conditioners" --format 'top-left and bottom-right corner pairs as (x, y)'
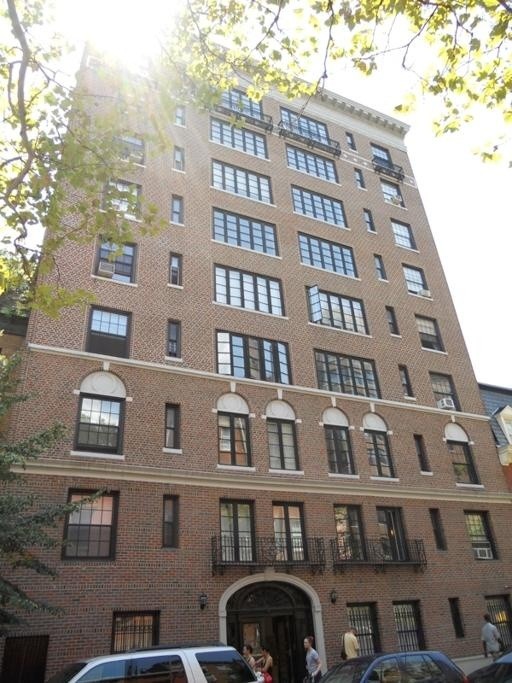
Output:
(95, 260), (115, 279)
(475, 548), (489, 559)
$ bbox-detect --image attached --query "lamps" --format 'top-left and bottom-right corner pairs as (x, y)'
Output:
(329, 587), (337, 603)
(198, 589), (209, 610)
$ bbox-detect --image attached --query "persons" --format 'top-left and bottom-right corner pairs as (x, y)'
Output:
(242, 644), (255, 673)
(481, 612), (506, 660)
(341, 626), (360, 659)
(303, 635), (323, 682)
(255, 641), (273, 683)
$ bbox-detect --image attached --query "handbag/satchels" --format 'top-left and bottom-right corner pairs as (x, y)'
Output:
(340, 646), (347, 660)
(262, 672), (273, 683)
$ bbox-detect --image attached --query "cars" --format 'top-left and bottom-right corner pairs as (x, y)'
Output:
(46, 646), (259, 683)
(317, 650), (512, 683)
